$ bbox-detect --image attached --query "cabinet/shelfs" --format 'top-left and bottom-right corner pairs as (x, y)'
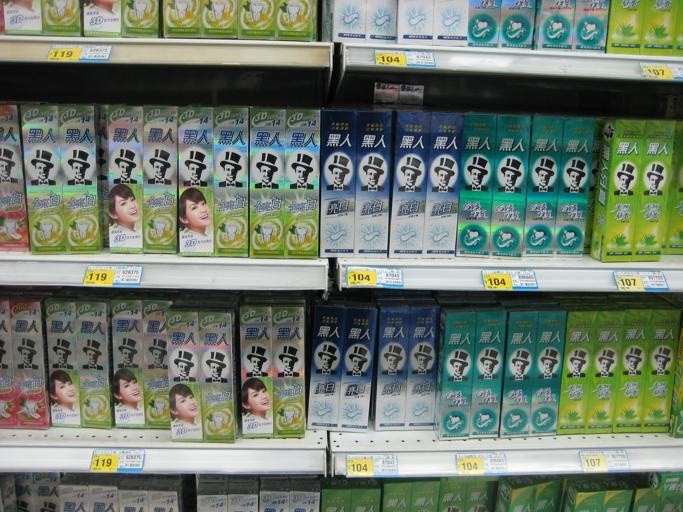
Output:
(0, 35), (335, 480)
(330, 41), (683, 480)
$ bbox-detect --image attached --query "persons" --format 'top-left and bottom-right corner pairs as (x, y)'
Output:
(179, 186), (215, 254)
(112, 370), (145, 427)
(170, 384), (203, 440)
(240, 379), (273, 436)
(2, 1), (41, 32)
(84, 0), (122, 33)
(47, 369), (79, 424)
(107, 184), (142, 247)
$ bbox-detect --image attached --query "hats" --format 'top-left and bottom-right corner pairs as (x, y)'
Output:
(617, 163), (635, 181)
(148, 338), (167, 356)
(67, 148), (91, 169)
(0, 148), (16, 167)
(246, 345), (267, 363)
(413, 345), (433, 361)
(449, 351), (470, 367)
(318, 344), (338, 361)
(30, 150), (54, 169)
(327, 154), (350, 175)
(466, 156), (489, 175)
(291, 153), (314, 173)
(278, 345), (299, 363)
(149, 149), (172, 169)
(569, 349), (588, 364)
(566, 159), (586, 178)
(535, 158), (555, 176)
(16, 337), (36, 356)
(624, 345), (644, 364)
(205, 351), (227, 369)
(52, 338), (72, 356)
(114, 149), (137, 169)
(82, 338), (102, 356)
(479, 348), (500, 365)
(118, 338), (138, 355)
(540, 348), (559, 364)
(597, 348), (616, 364)
(0, 339), (7, 355)
(348, 346), (368, 364)
(511, 350), (531, 367)
(401, 156), (422, 177)
(255, 152), (278, 173)
(499, 157), (523, 178)
(184, 150), (207, 170)
(173, 350), (194, 368)
(219, 151), (242, 172)
(362, 155), (385, 176)
(655, 347), (673, 362)
(434, 157), (456, 177)
(646, 163), (665, 181)
(383, 345), (404, 362)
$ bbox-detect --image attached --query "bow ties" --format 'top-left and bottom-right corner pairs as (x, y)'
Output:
(89, 366), (97, 369)
(406, 187), (415, 191)
(156, 180), (164, 184)
(650, 192), (657, 194)
(454, 378), (462, 381)
(1, 179), (10, 182)
(192, 182), (199, 185)
(226, 183), (235, 186)
(601, 373), (608, 377)
(658, 371), (665, 374)
(75, 181), (84, 184)
(369, 187), (376, 190)
(628, 372), (636, 375)
(155, 365), (163, 368)
(473, 186), (481, 190)
(298, 185), (306, 188)
(323, 371), (329, 373)
(439, 188), (447, 191)
(484, 376), (492, 379)
(539, 187), (547, 191)
(388, 371), (396, 375)
(515, 376), (523, 380)
(39, 181), (48, 184)
(334, 186), (342, 190)
(544, 375), (552, 378)
(570, 189), (578, 192)
(419, 371), (426, 373)
(180, 379), (188, 381)
(354, 373), (360, 375)
(24, 365), (32, 369)
(59, 365), (66, 369)
(121, 180), (129, 183)
(506, 188), (514, 192)
(253, 373), (260, 376)
(284, 374), (292, 376)
(574, 374), (580, 377)
(621, 191), (628, 194)
(213, 379), (220, 382)
(124, 365), (132, 368)
(262, 184), (271, 188)
